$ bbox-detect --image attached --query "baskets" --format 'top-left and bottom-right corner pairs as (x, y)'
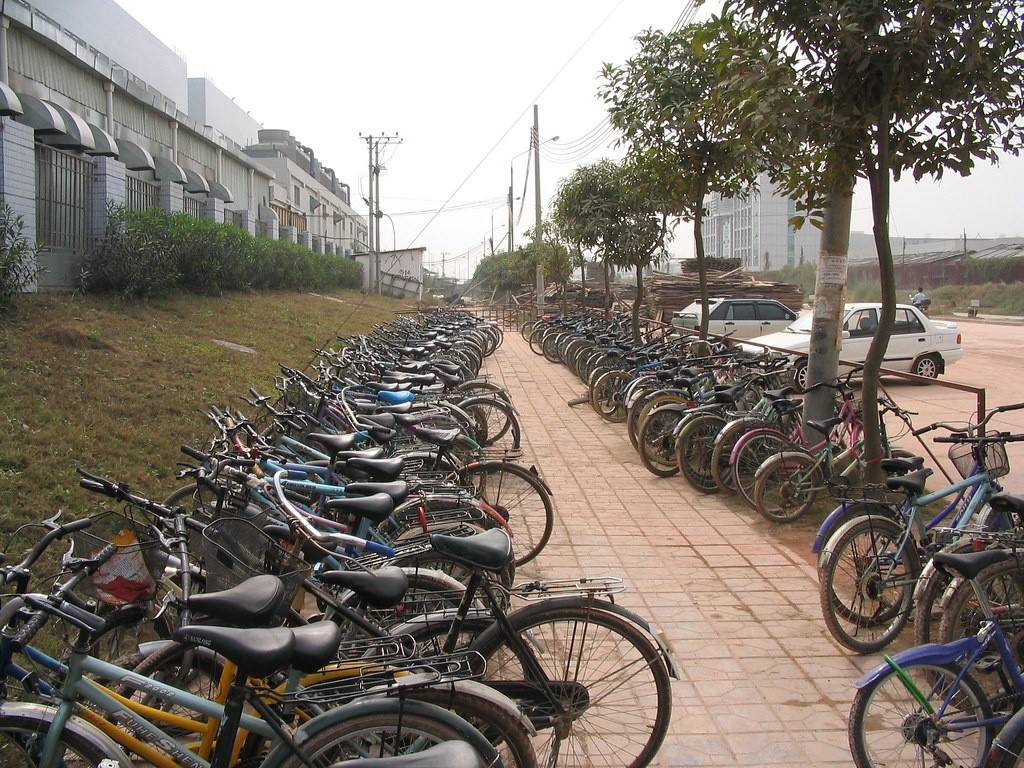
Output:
(189, 487), (272, 556)
(833, 387), (863, 417)
(948, 430), (1010, 480)
(286, 383), (316, 414)
(712, 338), (785, 384)
(73, 511), (170, 606)
(201, 518), (312, 628)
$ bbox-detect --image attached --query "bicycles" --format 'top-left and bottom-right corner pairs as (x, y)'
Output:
(0, 300), (1024, 768)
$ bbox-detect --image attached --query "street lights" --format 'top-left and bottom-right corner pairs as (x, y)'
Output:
(510, 135), (562, 253)
(484, 224), (506, 260)
(491, 196), (521, 256)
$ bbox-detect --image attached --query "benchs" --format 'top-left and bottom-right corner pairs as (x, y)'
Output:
(892, 320), (922, 334)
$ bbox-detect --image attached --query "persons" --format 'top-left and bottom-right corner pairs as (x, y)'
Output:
(799, 283), (804, 292)
(911, 287), (926, 305)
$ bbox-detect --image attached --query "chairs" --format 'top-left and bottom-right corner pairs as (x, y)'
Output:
(857, 317), (873, 336)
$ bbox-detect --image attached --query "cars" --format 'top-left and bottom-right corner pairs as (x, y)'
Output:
(671, 293), (802, 355)
(731, 302), (963, 393)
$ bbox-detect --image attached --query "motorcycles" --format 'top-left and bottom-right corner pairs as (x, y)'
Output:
(907, 294), (932, 321)
